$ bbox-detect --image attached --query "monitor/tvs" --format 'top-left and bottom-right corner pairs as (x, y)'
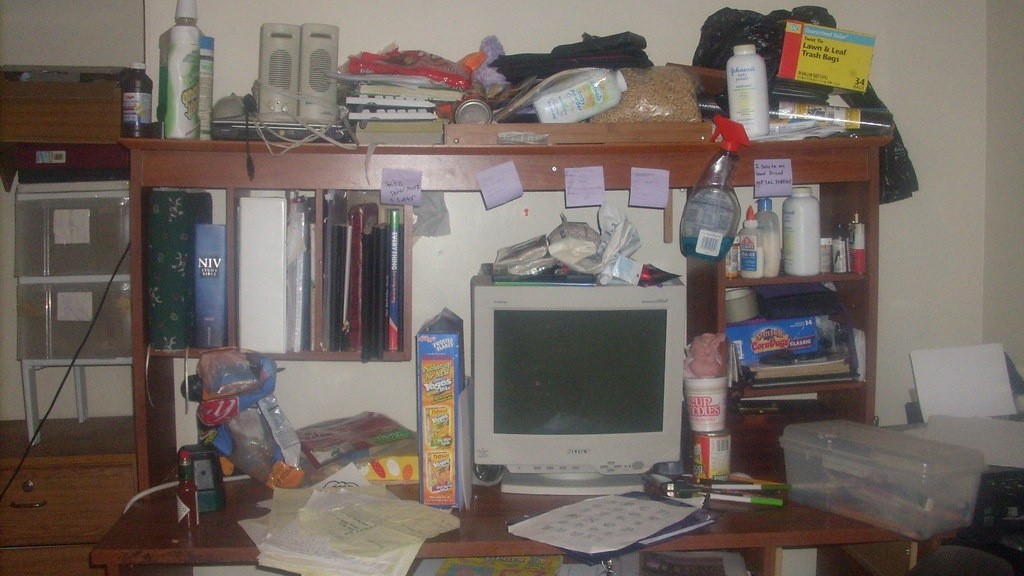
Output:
(468, 274), (687, 495)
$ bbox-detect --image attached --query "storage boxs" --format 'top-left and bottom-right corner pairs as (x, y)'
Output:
(776, 418), (986, 542)
(416, 304), (468, 511)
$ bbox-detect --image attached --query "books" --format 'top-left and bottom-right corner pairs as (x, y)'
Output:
(506, 490), (715, 566)
(236, 196), (315, 354)
(357, 84), (463, 101)
(324, 196), (403, 362)
(355, 120), (443, 145)
(146, 191), (226, 351)
(748, 356), (861, 389)
(739, 392), (818, 400)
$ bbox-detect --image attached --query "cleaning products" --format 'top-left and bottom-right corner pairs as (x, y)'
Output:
(678, 113), (753, 263)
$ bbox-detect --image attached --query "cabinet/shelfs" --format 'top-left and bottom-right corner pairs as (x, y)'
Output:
(0, 417), (138, 576)
(16, 178), (131, 367)
(715, 135), (888, 478)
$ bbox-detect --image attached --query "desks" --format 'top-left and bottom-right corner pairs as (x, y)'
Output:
(89, 461), (923, 576)
(118, 132), (892, 505)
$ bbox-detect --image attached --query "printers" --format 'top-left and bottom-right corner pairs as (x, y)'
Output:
(879, 354), (1024, 551)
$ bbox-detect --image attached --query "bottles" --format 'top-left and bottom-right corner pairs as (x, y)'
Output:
(753, 199), (780, 275)
(121, 63), (153, 137)
(725, 44), (770, 138)
(820, 238), (833, 273)
(531, 69), (627, 124)
(833, 224), (851, 273)
(176, 451), (199, 528)
(849, 211), (865, 273)
(162, 0), (214, 140)
(696, 91), (892, 135)
(740, 206), (764, 278)
(782, 187), (821, 276)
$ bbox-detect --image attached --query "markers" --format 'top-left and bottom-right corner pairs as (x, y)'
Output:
(711, 483), (785, 491)
(700, 492), (784, 506)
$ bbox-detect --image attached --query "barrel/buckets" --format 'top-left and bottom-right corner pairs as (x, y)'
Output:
(690, 432), (731, 486)
(682, 375), (728, 432)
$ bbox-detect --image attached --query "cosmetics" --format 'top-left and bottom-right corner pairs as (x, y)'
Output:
(726, 45), (770, 137)
(782, 187), (820, 275)
(534, 69), (627, 124)
(739, 198), (780, 278)
(818, 209), (866, 271)
